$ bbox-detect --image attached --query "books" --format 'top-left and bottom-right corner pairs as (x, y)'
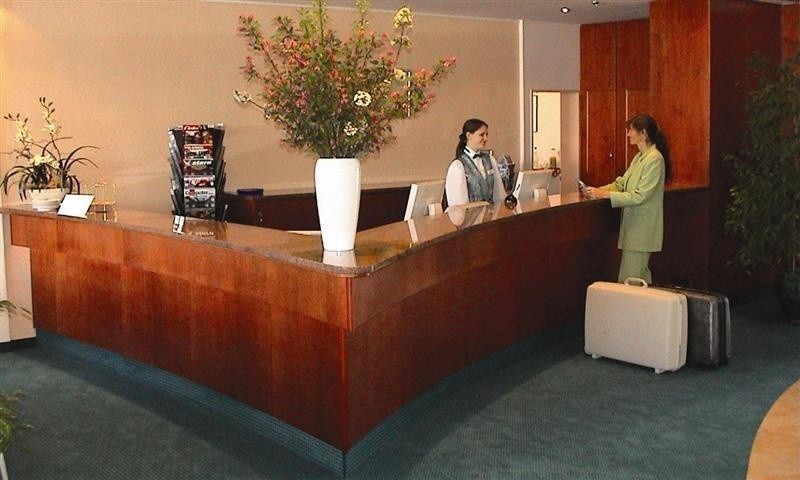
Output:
(167, 121), (228, 227)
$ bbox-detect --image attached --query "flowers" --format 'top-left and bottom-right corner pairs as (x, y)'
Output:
(0, 96), (101, 197)
(231, 0), (460, 159)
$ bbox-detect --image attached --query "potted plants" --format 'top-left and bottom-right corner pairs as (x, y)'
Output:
(711, 44), (800, 328)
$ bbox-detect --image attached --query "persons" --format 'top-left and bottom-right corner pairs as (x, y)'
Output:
(445, 118), (509, 208)
(582, 113), (670, 285)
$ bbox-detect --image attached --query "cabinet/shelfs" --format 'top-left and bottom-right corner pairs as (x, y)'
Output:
(578, 18), (650, 192)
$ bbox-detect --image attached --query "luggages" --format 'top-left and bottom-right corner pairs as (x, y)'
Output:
(583, 276), (689, 375)
(646, 282), (733, 366)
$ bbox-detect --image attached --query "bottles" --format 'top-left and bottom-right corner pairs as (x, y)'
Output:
(549, 148), (556, 168)
(505, 195), (517, 210)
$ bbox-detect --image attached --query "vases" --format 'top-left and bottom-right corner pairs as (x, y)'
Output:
(27, 188), (67, 212)
(314, 158), (362, 252)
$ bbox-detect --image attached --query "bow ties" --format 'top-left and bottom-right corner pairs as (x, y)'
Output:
(473, 154), (482, 160)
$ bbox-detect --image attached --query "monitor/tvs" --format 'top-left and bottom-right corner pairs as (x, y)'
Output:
(513, 169), (553, 199)
(408, 212), (458, 245)
(403, 179), (444, 222)
(516, 197), (551, 214)
